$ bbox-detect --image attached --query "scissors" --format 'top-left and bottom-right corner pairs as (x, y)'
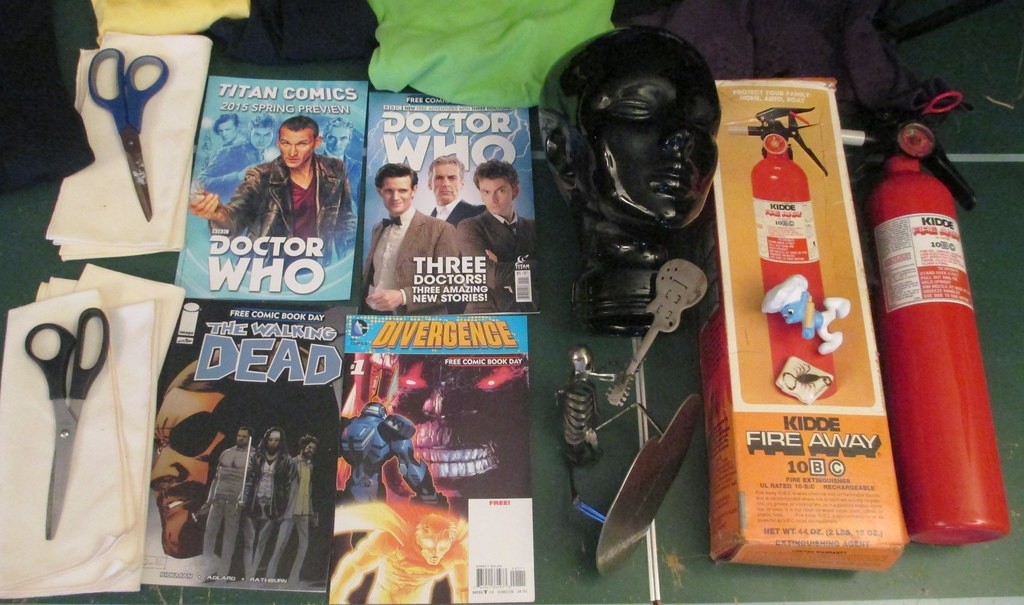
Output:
(87, 48), (170, 221)
(25, 308), (110, 541)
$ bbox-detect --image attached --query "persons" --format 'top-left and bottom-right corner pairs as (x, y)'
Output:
(541, 26), (723, 336)
(366, 154), (538, 313)
(187, 112), (354, 277)
(200, 426), (319, 586)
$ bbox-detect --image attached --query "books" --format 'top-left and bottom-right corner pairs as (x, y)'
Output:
(140, 298), (358, 592)
(328, 315), (536, 605)
(175, 75), (369, 301)
(363, 92), (540, 314)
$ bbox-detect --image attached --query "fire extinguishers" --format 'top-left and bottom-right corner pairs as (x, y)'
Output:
(725, 108), (838, 400)
(856, 116), (1010, 546)
